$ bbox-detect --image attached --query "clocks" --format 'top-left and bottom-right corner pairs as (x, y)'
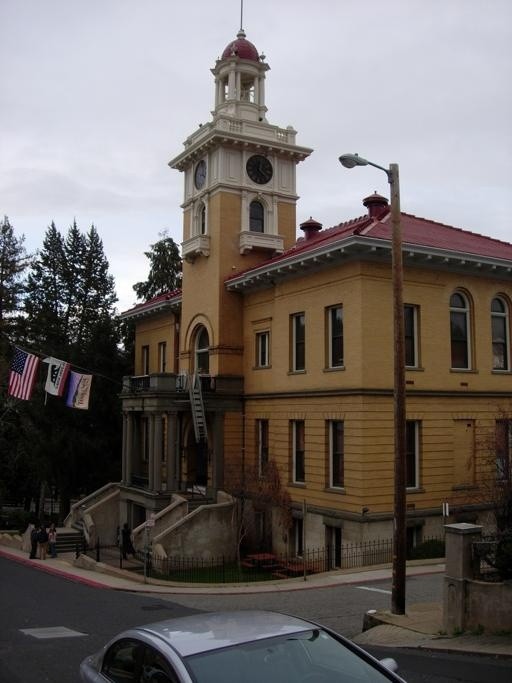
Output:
(244, 154), (273, 184)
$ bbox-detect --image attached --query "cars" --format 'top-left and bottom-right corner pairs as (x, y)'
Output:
(80, 608), (407, 682)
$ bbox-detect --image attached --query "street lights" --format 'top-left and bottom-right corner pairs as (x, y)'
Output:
(338, 151), (407, 614)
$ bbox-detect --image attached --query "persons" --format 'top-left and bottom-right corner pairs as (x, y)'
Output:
(36, 525), (48, 560)
(48, 529), (58, 558)
(49, 523), (57, 533)
(121, 523), (136, 560)
(29, 525), (40, 559)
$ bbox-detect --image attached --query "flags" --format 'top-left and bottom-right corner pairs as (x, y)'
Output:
(7, 347), (39, 400)
(65, 371), (94, 410)
(44, 357), (71, 397)
